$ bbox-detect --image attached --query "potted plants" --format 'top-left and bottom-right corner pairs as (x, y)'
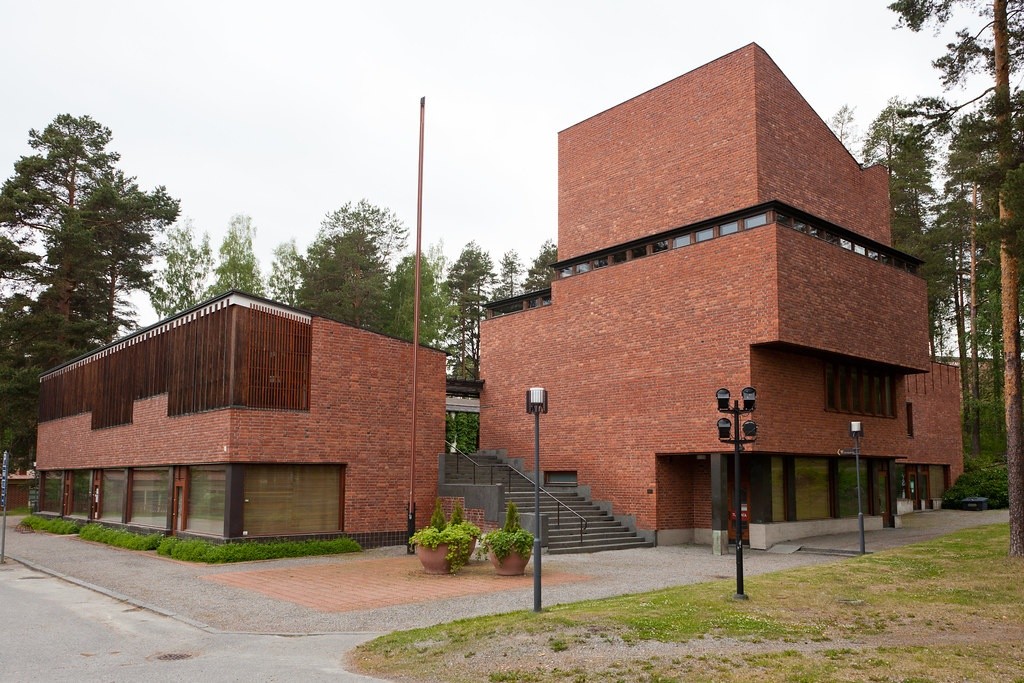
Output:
(408, 497), (482, 575)
(481, 501), (534, 575)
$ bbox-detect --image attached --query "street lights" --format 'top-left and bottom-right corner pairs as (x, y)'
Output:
(716, 386), (758, 597)
(849, 421), (865, 555)
(526, 389), (548, 610)
